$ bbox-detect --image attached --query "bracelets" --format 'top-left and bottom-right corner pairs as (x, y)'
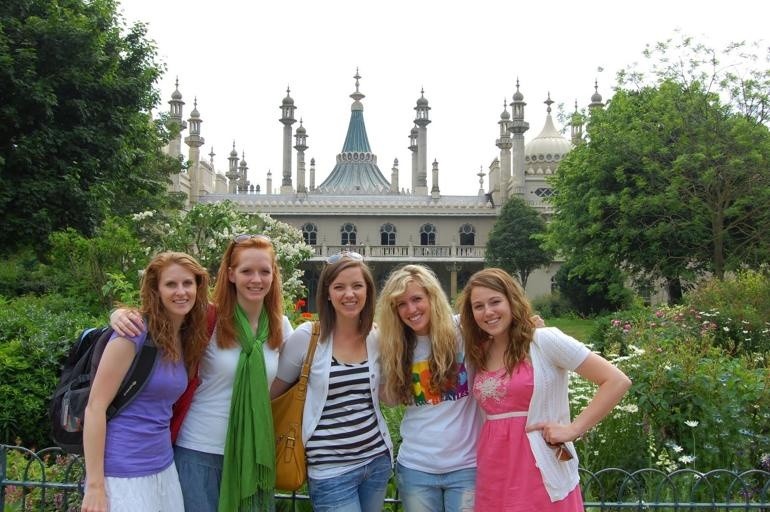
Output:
(109, 308), (117, 318)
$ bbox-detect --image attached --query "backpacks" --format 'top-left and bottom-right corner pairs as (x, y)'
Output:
(50, 322), (158, 448)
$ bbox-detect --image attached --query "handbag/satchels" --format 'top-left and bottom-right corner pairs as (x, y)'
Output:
(272, 379), (307, 491)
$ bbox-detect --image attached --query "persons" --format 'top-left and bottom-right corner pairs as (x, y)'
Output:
(110, 235), (293, 512)
(378, 263), (544, 512)
(270, 251), (398, 512)
(456, 267), (632, 512)
(80, 252), (210, 512)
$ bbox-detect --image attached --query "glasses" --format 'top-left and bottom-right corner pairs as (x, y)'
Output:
(324, 251), (362, 264)
(547, 442), (573, 461)
(234, 234), (271, 243)
